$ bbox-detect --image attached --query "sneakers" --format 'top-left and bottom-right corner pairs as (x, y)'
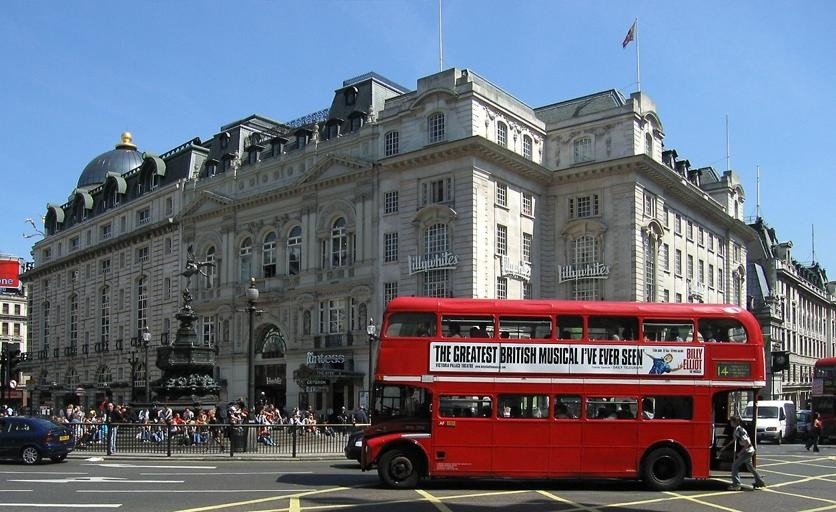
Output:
(752, 483), (766, 487)
(727, 484), (741, 488)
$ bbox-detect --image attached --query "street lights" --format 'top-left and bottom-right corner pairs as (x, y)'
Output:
(143, 325), (152, 402)
(365, 316), (377, 423)
(247, 275), (259, 425)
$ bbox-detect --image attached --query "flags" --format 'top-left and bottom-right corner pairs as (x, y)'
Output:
(622, 21), (635, 47)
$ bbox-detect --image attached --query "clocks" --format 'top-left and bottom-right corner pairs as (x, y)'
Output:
(772, 350), (790, 371)
(7, 378), (18, 391)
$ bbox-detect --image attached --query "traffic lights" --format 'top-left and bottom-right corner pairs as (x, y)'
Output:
(8, 343), (21, 384)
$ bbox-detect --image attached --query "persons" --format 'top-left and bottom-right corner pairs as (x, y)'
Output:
(805, 412), (823, 453)
(719, 415), (766, 490)
(1, 398), (370, 457)
(416, 318), (730, 344)
(437, 394), (658, 421)
(643, 349), (686, 376)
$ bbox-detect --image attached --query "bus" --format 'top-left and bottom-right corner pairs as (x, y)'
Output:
(345, 294), (766, 489)
(805, 357), (836, 444)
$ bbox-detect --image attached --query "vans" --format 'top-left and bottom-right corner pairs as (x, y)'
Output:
(742, 400), (795, 444)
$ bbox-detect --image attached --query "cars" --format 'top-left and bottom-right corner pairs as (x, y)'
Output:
(0, 415), (74, 465)
(796, 409), (812, 437)
(343, 430), (364, 463)
